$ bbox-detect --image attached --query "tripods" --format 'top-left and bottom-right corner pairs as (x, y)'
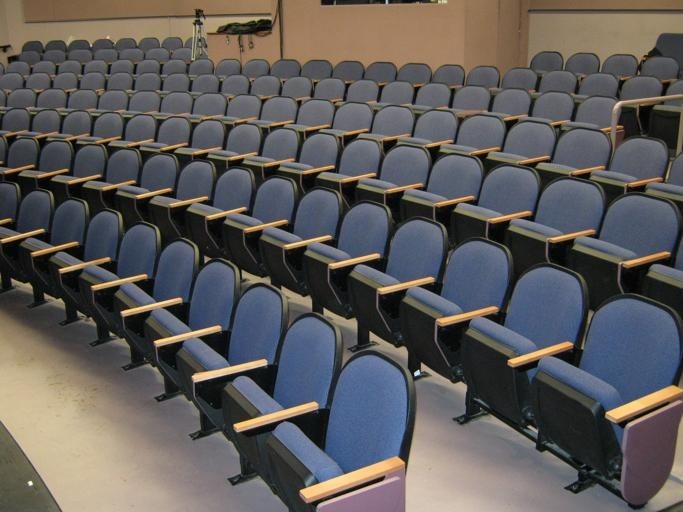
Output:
(190, 20), (207, 61)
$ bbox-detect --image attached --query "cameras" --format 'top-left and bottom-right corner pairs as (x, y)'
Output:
(194, 8), (206, 20)
